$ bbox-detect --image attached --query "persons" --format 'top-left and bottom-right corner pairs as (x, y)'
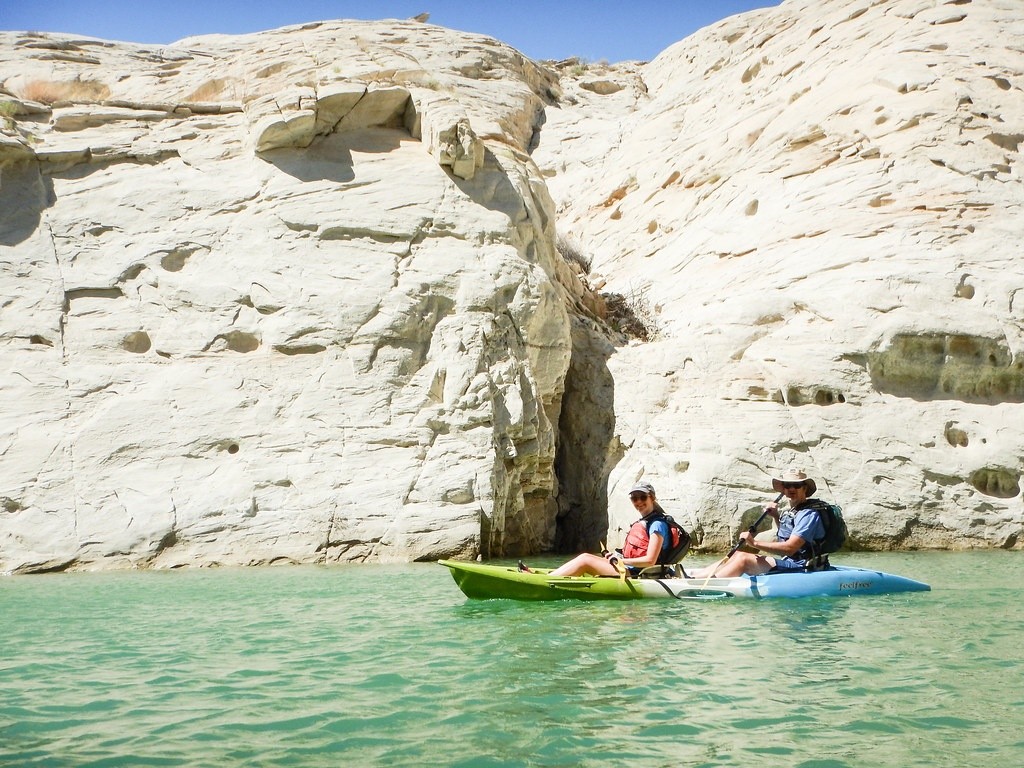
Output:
(517, 481), (672, 579)
(674, 467), (828, 579)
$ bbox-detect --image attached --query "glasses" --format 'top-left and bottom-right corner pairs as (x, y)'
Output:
(783, 483), (806, 489)
(630, 495), (652, 502)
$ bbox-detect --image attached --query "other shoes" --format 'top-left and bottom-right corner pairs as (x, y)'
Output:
(518, 560), (528, 573)
(675, 564), (695, 579)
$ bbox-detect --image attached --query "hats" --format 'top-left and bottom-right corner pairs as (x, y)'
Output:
(772, 472), (816, 497)
(628, 482), (655, 493)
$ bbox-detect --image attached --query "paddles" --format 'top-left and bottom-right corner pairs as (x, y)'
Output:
(599, 540), (629, 583)
(700, 492), (785, 592)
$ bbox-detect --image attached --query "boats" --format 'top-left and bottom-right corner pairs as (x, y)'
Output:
(435, 555), (934, 607)
